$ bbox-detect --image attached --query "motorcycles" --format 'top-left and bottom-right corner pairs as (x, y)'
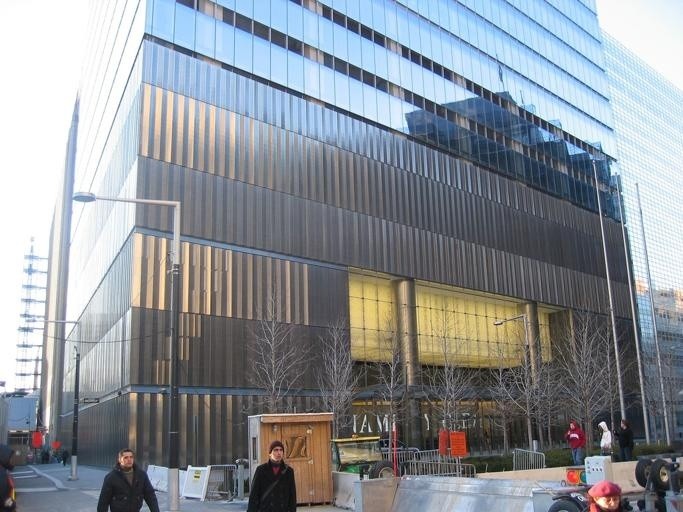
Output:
(543, 452), (683, 512)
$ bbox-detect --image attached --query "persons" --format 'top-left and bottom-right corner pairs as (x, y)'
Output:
(41, 450), (49, 464)
(564, 420), (586, 465)
(598, 420), (612, 456)
(588, 480), (622, 512)
(619, 420), (634, 461)
(97, 448), (160, 512)
(247, 441), (296, 512)
(0, 445), (16, 512)
(62, 450), (68, 466)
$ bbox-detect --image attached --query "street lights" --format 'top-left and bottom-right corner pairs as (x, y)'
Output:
(72, 192), (181, 511)
(494, 314), (535, 465)
(26, 319), (80, 481)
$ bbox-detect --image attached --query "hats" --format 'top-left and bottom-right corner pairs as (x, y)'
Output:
(268, 440), (285, 455)
(587, 481), (622, 498)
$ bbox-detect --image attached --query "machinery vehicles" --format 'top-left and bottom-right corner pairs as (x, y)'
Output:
(332, 434), (400, 486)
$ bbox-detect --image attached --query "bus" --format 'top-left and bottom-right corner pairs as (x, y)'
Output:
(7, 429), (35, 468)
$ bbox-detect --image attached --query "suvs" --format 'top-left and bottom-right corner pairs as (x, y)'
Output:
(375, 438), (422, 462)
